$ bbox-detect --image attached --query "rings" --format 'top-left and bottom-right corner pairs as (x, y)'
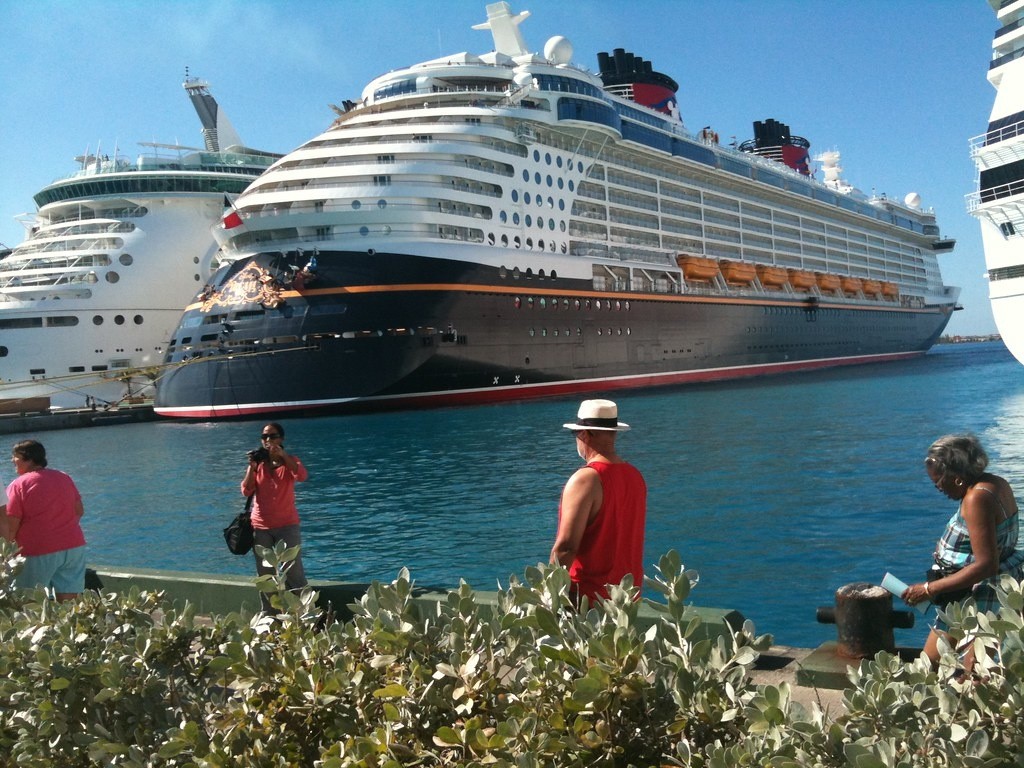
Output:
(907, 599), (913, 603)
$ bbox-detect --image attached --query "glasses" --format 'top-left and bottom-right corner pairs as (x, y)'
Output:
(571, 428), (593, 436)
(260, 432), (280, 439)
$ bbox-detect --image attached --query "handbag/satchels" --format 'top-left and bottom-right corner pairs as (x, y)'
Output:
(223, 512), (255, 554)
(926, 564), (973, 604)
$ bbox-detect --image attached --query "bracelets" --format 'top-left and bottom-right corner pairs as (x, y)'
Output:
(924, 581), (934, 600)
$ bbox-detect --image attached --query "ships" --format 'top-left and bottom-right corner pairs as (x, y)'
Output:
(967, 1), (1024, 368)
(1, 62), (284, 423)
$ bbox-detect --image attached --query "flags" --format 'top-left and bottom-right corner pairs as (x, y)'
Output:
(223, 212), (243, 229)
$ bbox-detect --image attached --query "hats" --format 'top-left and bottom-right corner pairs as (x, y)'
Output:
(564, 399), (633, 433)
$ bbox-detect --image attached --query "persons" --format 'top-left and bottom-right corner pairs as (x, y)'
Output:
(241, 422), (308, 615)
(105, 154), (109, 161)
(900, 432), (1023, 678)
(0, 470), (10, 541)
(5, 440), (86, 604)
(545, 399), (648, 619)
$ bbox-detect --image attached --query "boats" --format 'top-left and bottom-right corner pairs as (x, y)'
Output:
(786, 269), (817, 292)
(677, 254), (720, 283)
(839, 277), (863, 296)
(879, 282), (898, 297)
(862, 280), (881, 294)
(151, 0), (964, 423)
(815, 273), (841, 294)
(717, 260), (756, 287)
(756, 265), (787, 290)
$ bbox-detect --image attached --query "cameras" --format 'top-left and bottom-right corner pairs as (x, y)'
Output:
(250, 448), (272, 462)
